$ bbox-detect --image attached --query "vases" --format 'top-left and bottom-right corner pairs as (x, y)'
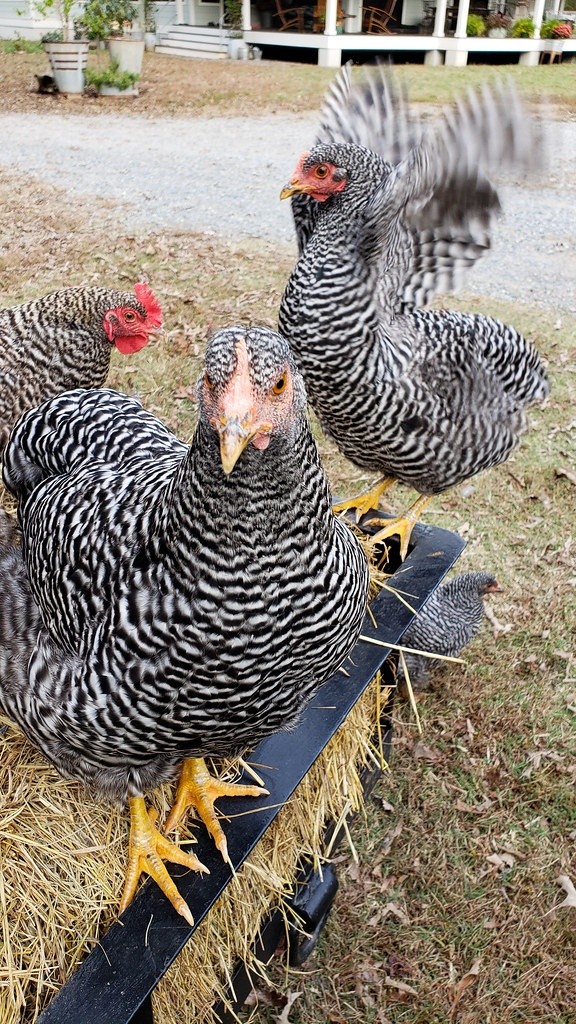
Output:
(545, 39), (565, 52)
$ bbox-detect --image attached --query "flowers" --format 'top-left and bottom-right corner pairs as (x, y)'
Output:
(551, 25), (572, 39)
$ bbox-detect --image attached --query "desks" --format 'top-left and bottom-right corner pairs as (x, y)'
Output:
(429, 6), (494, 34)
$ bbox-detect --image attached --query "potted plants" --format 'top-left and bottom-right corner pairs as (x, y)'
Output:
(143, 0), (159, 52)
(467, 14), (563, 38)
(225, 0), (247, 59)
(16, 0), (144, 96)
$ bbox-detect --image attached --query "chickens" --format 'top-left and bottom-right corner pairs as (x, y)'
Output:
(388, 569), (501, 708)
(1, 281), (166, 469)
(0, 321), (373, 930)
(272, 53), (559, 567)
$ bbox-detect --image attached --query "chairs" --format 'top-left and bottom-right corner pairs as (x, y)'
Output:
(272, 0), (309, 31)
(361, 0), (397, 36)
